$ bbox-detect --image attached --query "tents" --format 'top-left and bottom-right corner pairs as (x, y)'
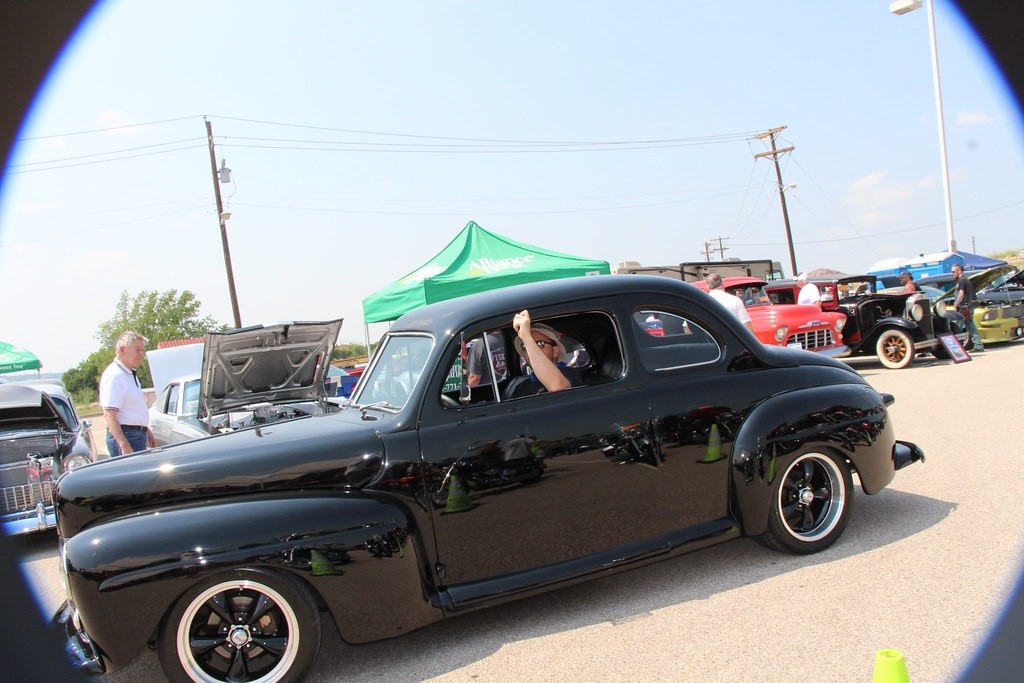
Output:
(0, 341), (42, 377)
(362, 220), (610, 390)
(945, 249), (1006, 270)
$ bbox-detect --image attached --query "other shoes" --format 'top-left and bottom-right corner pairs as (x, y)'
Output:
(969, 348), (984, 353)
(918, 354), (929, 357)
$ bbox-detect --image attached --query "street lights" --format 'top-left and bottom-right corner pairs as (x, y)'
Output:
(780, 184), (798, 277)
(212, 162), (243, 330)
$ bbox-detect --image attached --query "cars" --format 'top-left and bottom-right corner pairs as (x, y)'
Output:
(145, 319), (346, 449)
(935, 268), (1024, 307)
(876, 266), (1024, 351)
(0, 385), (98, 538)
(643, 277), (851, 359)
(51, 274), (925, 683)
(759, 275), (970, 370)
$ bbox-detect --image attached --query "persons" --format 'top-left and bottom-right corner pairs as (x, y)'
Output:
(797, 274), (822, 310)
(661, 315), (701, 335)
(99, 331), (156, 457)
(735, 288), (760, 305)
(897, 271), (921, 293)
(502, 434), (542, 479)
(706, 273), (753, 333)
(951, 265), (984, 353)
(466, 330), (505, 387)
(505, 309), (582, 400)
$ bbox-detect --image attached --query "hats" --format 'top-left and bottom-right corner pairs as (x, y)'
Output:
(897, 270), (912, 278)
(514, 323), (566, 363)
(706, 274), (725, 288)
(798, 272), (809, 283)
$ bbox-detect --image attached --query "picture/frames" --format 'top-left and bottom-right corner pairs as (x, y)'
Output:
(936, 332), (972, 363)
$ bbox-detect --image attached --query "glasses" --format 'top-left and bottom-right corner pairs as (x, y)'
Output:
(521, 341), (553, 351)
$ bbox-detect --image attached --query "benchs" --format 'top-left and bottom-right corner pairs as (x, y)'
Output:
(596, 344), (717, 380)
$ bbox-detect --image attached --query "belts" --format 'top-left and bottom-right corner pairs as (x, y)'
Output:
(120, 425), (148, 432)
